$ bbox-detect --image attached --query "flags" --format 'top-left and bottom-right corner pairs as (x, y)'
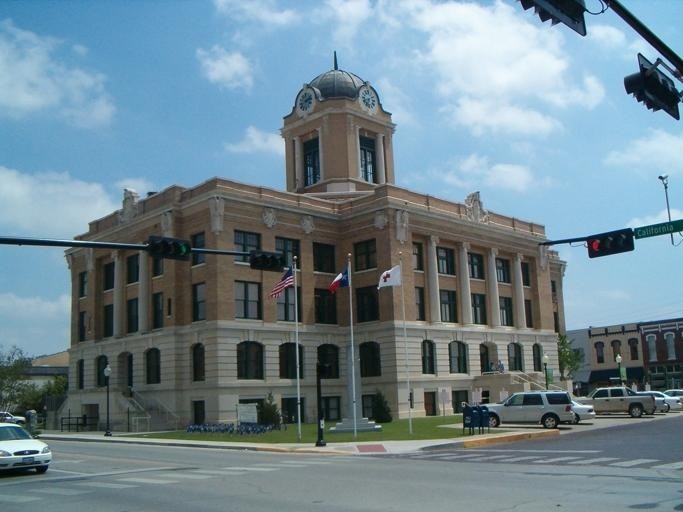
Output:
(272, 264), (294, 298)
(376, 264), (401, 290)
(328, 264), (349, 294)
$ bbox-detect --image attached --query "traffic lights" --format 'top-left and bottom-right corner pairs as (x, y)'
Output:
(588, 227), (635, 262)
(249, 249), (285, 274)
(319, 363), (332, 377)
(518, 0), (587, 36)
(621, 52), (680, 120)
(148, 235), (191, 262)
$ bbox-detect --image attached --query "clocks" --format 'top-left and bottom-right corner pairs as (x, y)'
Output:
(294, 83), (317, 117)
(358, 81), (378, 114)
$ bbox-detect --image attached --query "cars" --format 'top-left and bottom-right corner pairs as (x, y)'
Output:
(0, 422), (51, 474)
(571, 399), (598, 423)
(662, 388), (683, 401)
(641, 391), (668, 415)
(636, 390), (683, 412)
(0, 411), (25, 425)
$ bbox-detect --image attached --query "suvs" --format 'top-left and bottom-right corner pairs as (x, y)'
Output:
(471, 387), (575, 430)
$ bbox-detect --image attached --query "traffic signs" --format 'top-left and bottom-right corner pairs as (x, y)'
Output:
(634, 218), (683, 239)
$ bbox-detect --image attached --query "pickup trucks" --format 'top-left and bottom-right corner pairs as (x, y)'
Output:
(573, 385), (658, 418)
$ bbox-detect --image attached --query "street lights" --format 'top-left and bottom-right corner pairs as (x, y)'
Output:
(103, 362), (112, 436)
(615, 353), (623, 385)
(541, 352), (550, 390)
(42, 404), (47, 430)
